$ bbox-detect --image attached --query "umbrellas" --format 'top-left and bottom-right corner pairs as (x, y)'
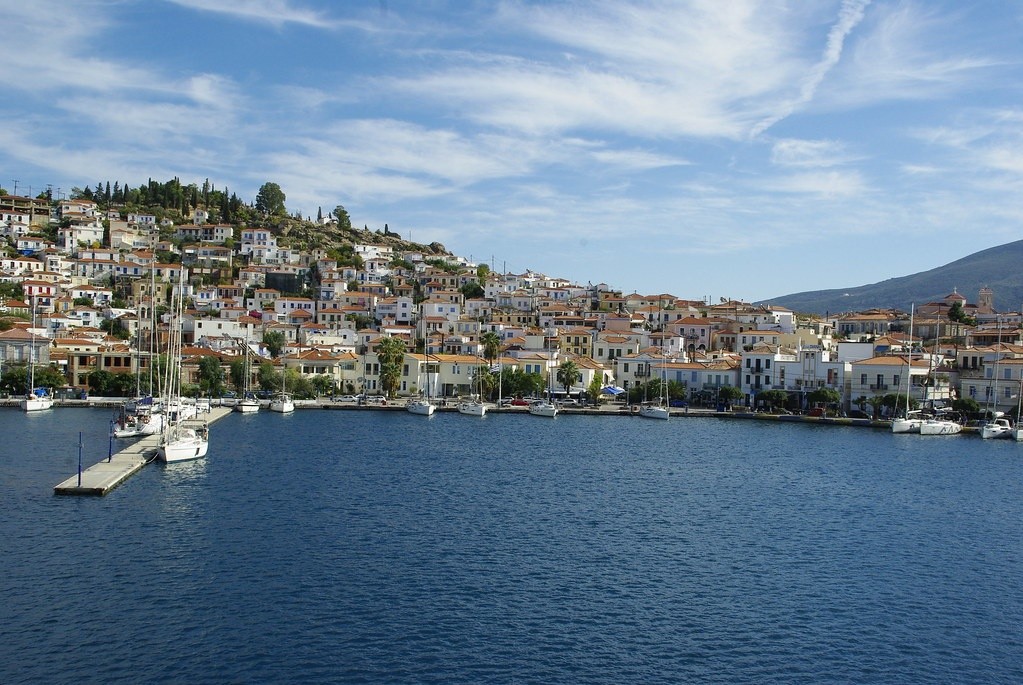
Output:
(601, 387), (625, 395)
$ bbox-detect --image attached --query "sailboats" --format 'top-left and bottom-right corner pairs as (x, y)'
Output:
(456, 318), (486, 417)
(110, 254), (212, 437)
(270, 311), (295, 413)
(155, 260), (209, 462)
(234, 324), (261, 412)
(528, 325), (557, 417)
(1012, 375), (1023, 442)
(891, 303), (926, 435)
(638, 324), (670, 420)
(405, 313), (437, 416)
(980, 319), (1013, 440)
(918, 314), (965, 435)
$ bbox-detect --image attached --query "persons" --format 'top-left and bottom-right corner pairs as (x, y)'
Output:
(118, 413), (150, 428)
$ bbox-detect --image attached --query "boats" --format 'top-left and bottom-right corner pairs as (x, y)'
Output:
(20, 287), (55, 412)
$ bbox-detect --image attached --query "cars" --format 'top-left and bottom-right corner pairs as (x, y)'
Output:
(899, 406), (967, 427)
(29, 389), (48, 397)
(496, 395), (579, 408)
(848, 410), (873, 420)
(222, 389), (273, 399)
(807, 408), (824, 417)
(670, 399), (688, 407)
(716, 402), (803, 415)
(329, 394), (387, 404)
